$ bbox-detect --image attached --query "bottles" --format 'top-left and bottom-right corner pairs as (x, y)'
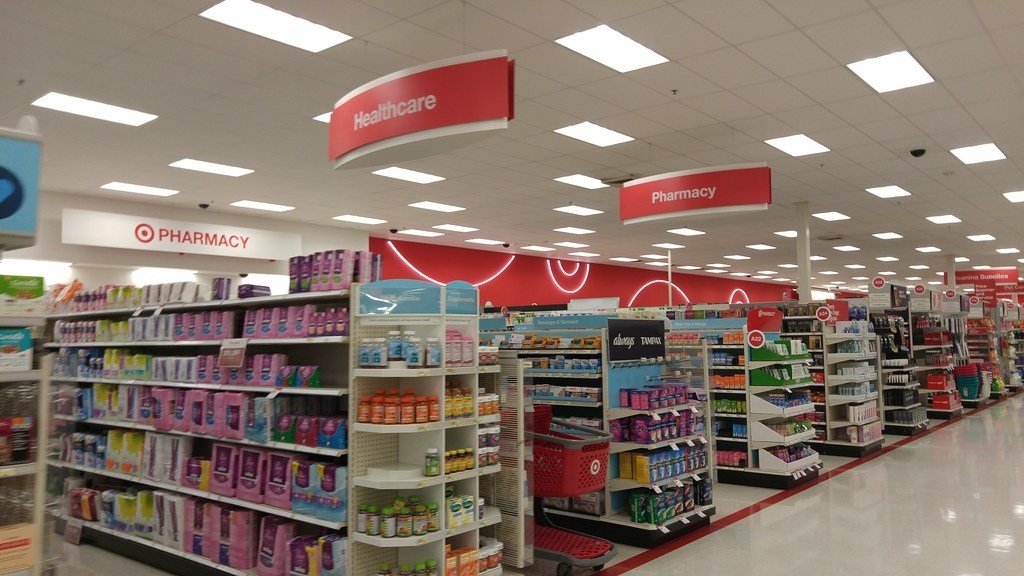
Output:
(401, 331), (417, 361)
(425, 337), (442, 369)
(358, 388), (440, 425)
(372, 337), (388, 368)
(386, 330), (402, 361)
(406, 338), (424, 369)
(424, 448), (441, 477)
(355, 496), (440, 538)
(359, 338), (374, 369)
(445, 447), (475, 475)
(358, 559), (439, 576)
(445, 379), (475, 418)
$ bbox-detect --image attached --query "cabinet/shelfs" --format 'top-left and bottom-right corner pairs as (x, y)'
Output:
(878, 366), (929, 436)
(43, 280), (503, 576)
(672, 318), (823, 490)
(0, 352), (57, 576)
(479, 320), (716, 549)
(909, 310), (963, 420)
(784, 316), (885, 458)
(955, 319), (1024, 408)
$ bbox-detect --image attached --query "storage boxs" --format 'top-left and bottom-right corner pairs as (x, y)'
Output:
(923, 329), (962, 410)
(144, 249), (382, 576)
(616, 442), (707, 484)
